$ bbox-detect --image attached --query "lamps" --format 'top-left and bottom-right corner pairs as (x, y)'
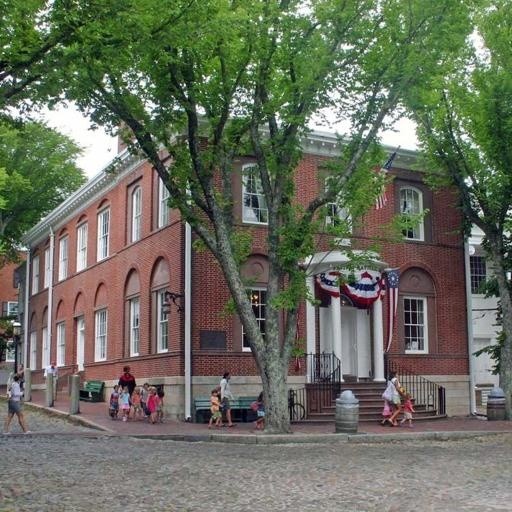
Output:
(162, 289), (185, 314)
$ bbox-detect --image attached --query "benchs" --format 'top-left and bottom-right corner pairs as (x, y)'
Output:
(80, 380), (105, 402)
(194, 395), (258, 423)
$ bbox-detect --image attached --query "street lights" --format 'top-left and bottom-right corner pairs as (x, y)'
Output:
(9, 318), (23, 374)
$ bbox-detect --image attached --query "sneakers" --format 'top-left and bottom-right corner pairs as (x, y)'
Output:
(381, 420), (416, 428)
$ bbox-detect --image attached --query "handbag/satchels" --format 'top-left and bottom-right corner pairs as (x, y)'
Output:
(392, 395), (401, 405)
(382, 391), (392, 402)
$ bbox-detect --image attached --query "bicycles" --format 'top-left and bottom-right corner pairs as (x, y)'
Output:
(285, 387), (306, 424)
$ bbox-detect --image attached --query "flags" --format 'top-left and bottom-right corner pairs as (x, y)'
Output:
(372, 145), (401, 211)
(313, 267), (385, 315)
(381, 265), (400, 354)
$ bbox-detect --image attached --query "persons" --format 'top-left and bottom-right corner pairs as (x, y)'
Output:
(1, 374), (33, 434)
(44, 360), (59, 401)
(207, 388), (224, 429)
(250, 391), (265, 430)
(382, 369), (408, 427)
(216, 371), (238, 428)
(17, 362), (24, 393)
(398, 392), (415, 427)
(380, 400), (399, 426)
(106, 365), (166, 424)
